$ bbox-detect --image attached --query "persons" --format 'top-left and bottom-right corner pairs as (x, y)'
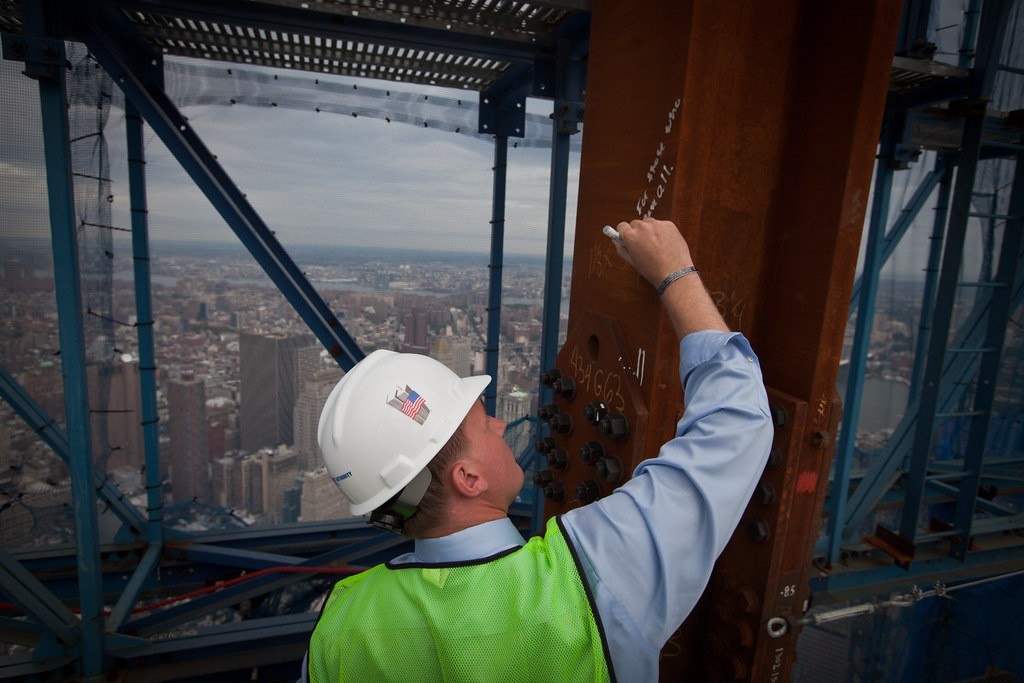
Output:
(293, 216), (776, 683)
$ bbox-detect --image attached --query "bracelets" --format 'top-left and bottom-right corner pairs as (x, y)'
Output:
(655, 266), (701, 302)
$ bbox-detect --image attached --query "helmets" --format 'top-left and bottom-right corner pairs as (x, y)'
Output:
(317, 349), (492, 516)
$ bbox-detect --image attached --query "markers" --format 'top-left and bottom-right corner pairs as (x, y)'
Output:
(600, 224), (628, 251)
